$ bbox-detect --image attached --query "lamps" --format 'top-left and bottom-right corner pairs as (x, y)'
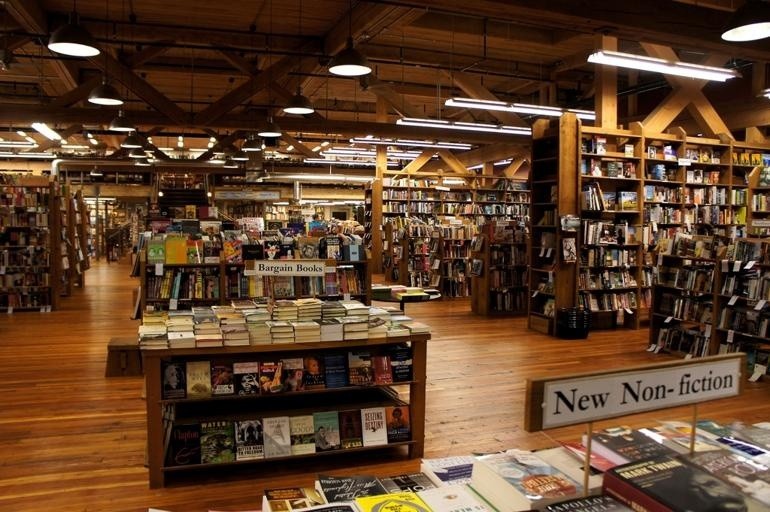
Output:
(587, 27), (743, 83)
(88, 40), (125, 106)
(48, 0), (102, 58)
(328, 0), (373, 77)
(721, 0), (770, 47)
(282, 53), (319, 115)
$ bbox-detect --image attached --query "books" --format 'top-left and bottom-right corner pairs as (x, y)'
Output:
(139, 296), (429, 351)
(657, 236), (769, 374)
(144, 217), (363, 308)
(536, 185), (556, 317)
(390, 225), (484, 299)
(364, 181), (531, 250)
(1, 168), (59, 311)
(159, 173), (265, 220)
(60, 197), (90, 288)
(641, 144), (770, 308)
(263, 421), (769, 512)
(109, 209), (140, 247)
(160, 343), (412, 464)
(580, 135), (640, 313)
(489, 227), (535, 312)
(266, 205), (323, 223)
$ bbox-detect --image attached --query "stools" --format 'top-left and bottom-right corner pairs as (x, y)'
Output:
(105, 337), (142, 377)
